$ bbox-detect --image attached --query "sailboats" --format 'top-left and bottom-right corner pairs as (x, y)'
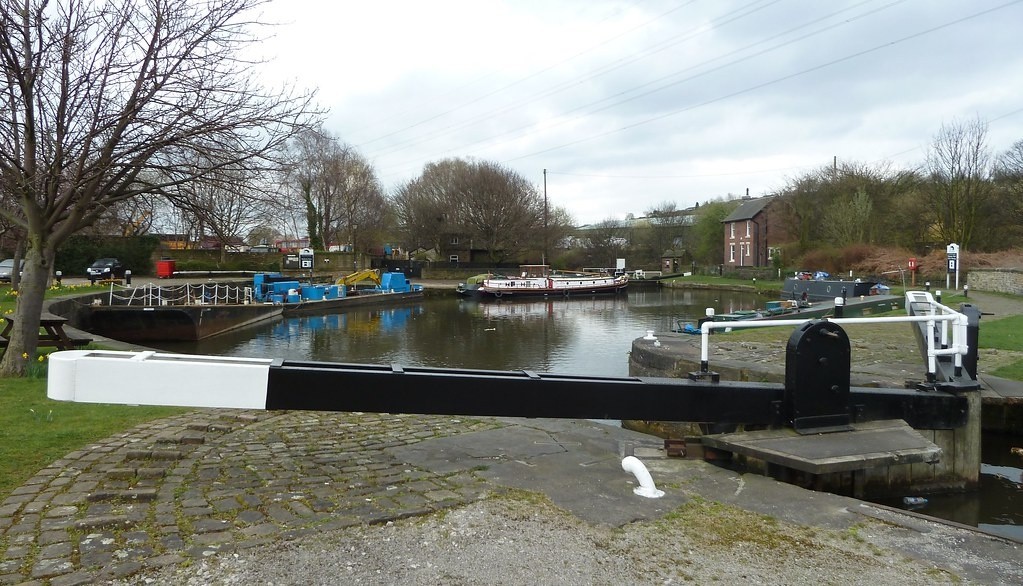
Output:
(476, 168), (631, 297)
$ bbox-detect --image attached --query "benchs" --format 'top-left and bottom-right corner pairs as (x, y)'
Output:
(55, 325), (94, 345)
(0, 335), (9, 349)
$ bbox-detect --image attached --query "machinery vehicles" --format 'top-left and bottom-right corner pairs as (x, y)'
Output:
(329, 268), (381, 297)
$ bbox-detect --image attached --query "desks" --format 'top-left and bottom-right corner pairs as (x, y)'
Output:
(1, 311), (74, 362)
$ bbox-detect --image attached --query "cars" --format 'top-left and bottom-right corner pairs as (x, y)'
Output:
(0, 259), (25, 282)
(86, 258), (125, 280)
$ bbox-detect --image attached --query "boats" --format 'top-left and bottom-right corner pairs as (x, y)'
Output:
(782, 270), (878, 301)
(457, 272), (594, 296)
(671, 293), (906, 334)
(252, 272), (426, 316)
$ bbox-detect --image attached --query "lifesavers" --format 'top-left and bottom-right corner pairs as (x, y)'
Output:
(563, 289), (570, 296)
(616, 286), (621, 294)
(495, 289), (503, 298)
